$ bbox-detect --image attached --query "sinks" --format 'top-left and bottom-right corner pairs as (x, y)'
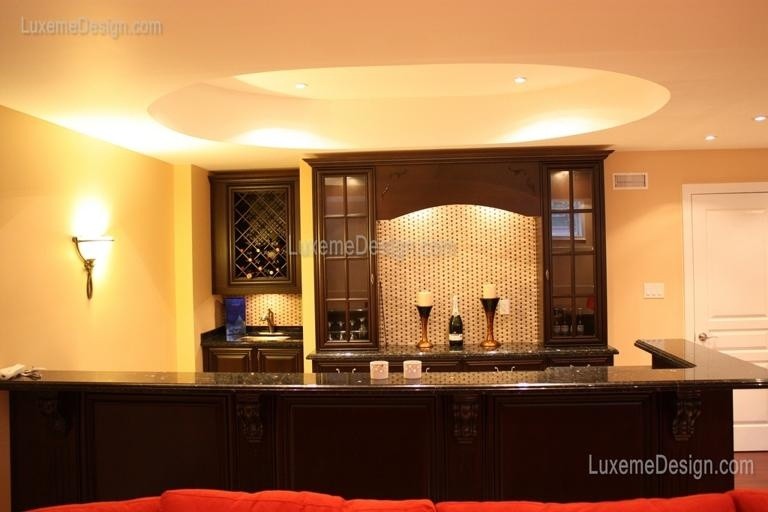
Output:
(235, 334), (292, 342)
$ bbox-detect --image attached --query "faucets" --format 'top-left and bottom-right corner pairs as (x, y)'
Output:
(260, 307), (276, 332)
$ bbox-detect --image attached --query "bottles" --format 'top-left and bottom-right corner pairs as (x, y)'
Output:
(238, 244), (282, 279)
(446, 295), (465, 351)
(553, 306), (587, 335)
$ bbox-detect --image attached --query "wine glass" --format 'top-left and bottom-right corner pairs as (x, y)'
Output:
(327, 318), (369, 339)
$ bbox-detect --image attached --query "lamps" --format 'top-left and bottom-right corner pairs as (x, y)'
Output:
(69, 198), (115, 300)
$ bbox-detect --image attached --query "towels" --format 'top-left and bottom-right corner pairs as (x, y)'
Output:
(403, 360), (421, 380)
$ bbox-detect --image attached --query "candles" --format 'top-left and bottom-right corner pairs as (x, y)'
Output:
(416, 291), (433, 306)
(483, 284), (497, 299)
(369, 359), (423, 380)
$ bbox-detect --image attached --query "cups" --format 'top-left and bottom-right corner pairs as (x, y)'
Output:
(402, 359), (423, 380)
(369, 360), (389, 380)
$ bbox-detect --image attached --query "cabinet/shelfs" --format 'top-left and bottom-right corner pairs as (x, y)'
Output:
(203, 346), (302, 373)
(311, 164), (378, 351)
(542, 161), (605, 345)
